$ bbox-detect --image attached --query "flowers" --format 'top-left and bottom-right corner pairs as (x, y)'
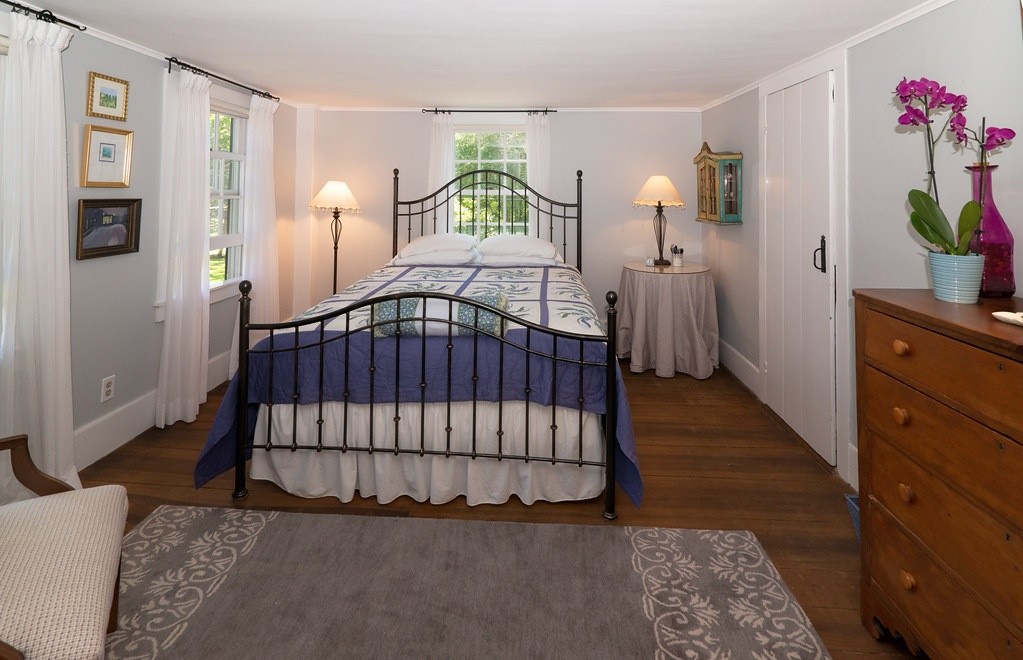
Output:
(893, 75), (1016, 256)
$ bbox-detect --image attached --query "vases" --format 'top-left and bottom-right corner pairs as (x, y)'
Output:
(927, 250), (986, 304)
(963, 164), (1016, 297)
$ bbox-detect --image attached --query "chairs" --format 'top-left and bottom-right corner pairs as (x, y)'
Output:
(0, 434), (130, 660)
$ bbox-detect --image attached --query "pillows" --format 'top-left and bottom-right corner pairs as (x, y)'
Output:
(386, 233), (565, 267)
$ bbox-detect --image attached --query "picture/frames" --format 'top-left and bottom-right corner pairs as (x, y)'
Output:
(75, 198), (142, 260)
(85, 70), (130, 121)
(79, 122), (134, 188)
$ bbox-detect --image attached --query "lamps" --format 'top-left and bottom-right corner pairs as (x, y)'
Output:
(631, 175), (688, 265)
(309, 181), (361, 297)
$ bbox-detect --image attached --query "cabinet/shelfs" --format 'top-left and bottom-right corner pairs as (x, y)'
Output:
(852, 286), (1023, 659)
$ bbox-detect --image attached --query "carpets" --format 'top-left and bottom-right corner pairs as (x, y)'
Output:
(102, 504), (834, 660)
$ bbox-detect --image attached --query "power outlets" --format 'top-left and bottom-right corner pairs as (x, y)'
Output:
(100, 374), (116, 403)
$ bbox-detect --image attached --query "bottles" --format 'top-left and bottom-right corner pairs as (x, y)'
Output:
(958, 162), (1016, 301)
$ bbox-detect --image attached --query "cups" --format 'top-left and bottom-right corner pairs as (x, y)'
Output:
(671, 253), (684, 266)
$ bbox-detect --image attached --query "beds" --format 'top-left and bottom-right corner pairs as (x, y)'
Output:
(194, 168), (644, 519)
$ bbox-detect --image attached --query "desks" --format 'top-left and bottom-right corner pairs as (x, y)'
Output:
(613, 262), (720, 379)
